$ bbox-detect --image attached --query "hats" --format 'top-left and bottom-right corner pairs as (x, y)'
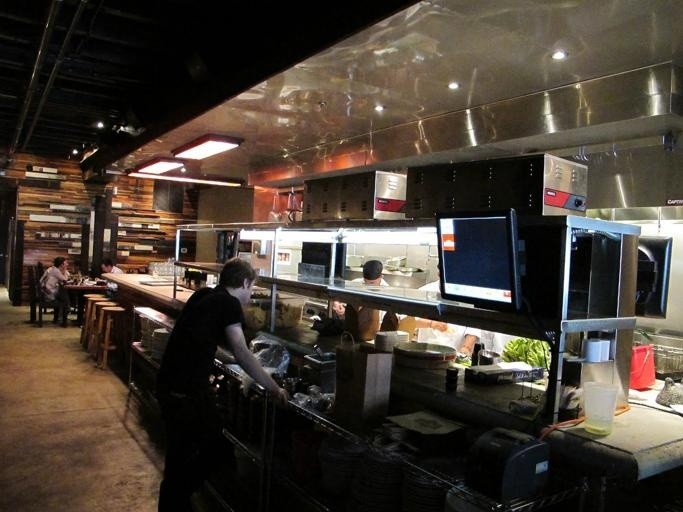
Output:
(363, 260), (383, 279)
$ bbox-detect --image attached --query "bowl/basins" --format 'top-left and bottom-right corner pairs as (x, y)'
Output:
(375, 332), (410, 352)
(386, 256), (407, 272)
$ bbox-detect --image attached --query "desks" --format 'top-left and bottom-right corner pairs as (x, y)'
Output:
(101, 273), (195, 383)
(546, 404), (682, 512)
(58, 280), (106, 328)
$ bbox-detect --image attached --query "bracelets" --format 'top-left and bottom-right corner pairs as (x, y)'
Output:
(463, 344), (472, 352)
(429, 320), (432, 328)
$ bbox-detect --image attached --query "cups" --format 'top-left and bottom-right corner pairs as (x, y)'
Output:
(582, 383), (619, 437)
(285, 377), (302, 396)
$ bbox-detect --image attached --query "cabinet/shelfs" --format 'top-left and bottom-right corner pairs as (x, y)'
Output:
(124, 305), (176, 409)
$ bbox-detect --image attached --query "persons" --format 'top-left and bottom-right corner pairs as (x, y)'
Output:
(92, 260), (126, 296)
(152, 255), (292, 512)
(38, 256), (74, 325)
(413, 261), (480, 362)
(331, 258), (406, 327)
(33, 260), (70, 297)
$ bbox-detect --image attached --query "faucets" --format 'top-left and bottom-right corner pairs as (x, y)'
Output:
(638, 328), (652, 340)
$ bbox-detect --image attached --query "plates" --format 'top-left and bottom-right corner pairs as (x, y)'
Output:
(386, 411), (464, 436)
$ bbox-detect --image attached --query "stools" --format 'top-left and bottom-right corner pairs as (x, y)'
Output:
(80, 294), (126, 370)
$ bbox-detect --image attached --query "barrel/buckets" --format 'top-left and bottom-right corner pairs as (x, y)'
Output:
(629, 345), (656, 389)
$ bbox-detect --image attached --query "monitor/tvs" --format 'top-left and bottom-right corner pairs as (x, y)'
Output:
(434, 207), (522, 314)
(302, 242), (347, 279)
(635, 235), (674, 319)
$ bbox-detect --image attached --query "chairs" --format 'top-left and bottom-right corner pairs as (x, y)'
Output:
(27, 262), (58, 327)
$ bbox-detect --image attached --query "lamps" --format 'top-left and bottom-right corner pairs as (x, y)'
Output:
(126, 134), (245, 188)
